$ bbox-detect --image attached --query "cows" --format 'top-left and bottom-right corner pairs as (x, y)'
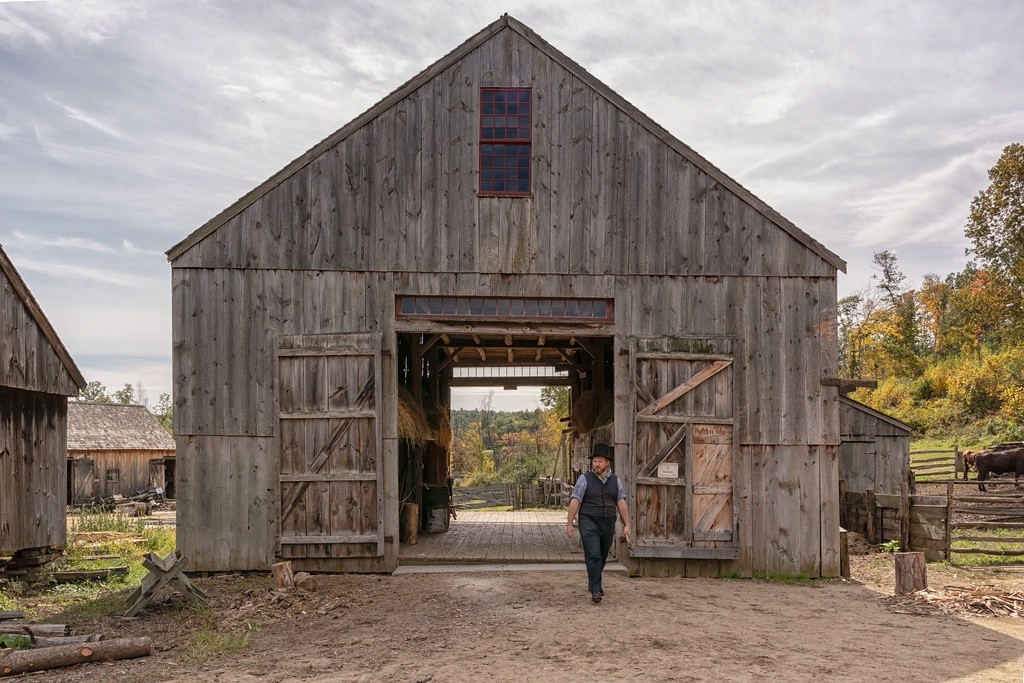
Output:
(961, 448), (1024, 492)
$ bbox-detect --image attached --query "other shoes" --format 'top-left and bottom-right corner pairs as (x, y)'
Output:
(592, 591), (602, 602)
(588, 587), (604, 596)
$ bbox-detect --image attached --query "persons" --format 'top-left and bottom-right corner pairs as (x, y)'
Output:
(565, 442), (630, 602)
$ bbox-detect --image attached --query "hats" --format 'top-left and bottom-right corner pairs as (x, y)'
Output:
(587, 444), (613, 461)
